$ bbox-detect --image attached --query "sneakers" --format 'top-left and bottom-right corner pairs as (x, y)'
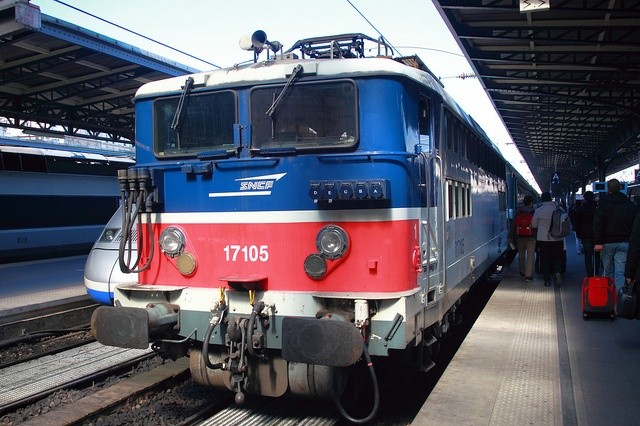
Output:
(519, 271), (524, 276)
(525, 276), (533, 282)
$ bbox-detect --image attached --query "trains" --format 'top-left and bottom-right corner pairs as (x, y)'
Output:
(91, 29), (540, 423)
(84, 196), (138, 306)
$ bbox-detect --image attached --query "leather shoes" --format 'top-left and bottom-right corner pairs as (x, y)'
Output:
(554, 272), (562, 287)
(544, 279), (551, 287)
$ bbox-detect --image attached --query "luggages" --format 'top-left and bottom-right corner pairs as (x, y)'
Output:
(581, 247), (617, 321)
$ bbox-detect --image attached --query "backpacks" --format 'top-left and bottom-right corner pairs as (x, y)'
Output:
(547, 205), (570, 239)
(516, 210), (533, 236)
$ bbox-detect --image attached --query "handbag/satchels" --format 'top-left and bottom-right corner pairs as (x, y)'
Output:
(617, 279), (640, 321)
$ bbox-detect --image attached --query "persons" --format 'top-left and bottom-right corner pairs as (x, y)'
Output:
(591, 179), (636, 293)
(508, 195), (537, 281)
(575, 191), (597, 254)
(530, 192), (571, 287)
(570, 199), (582, 225)
(623, 211), (640, 320)
